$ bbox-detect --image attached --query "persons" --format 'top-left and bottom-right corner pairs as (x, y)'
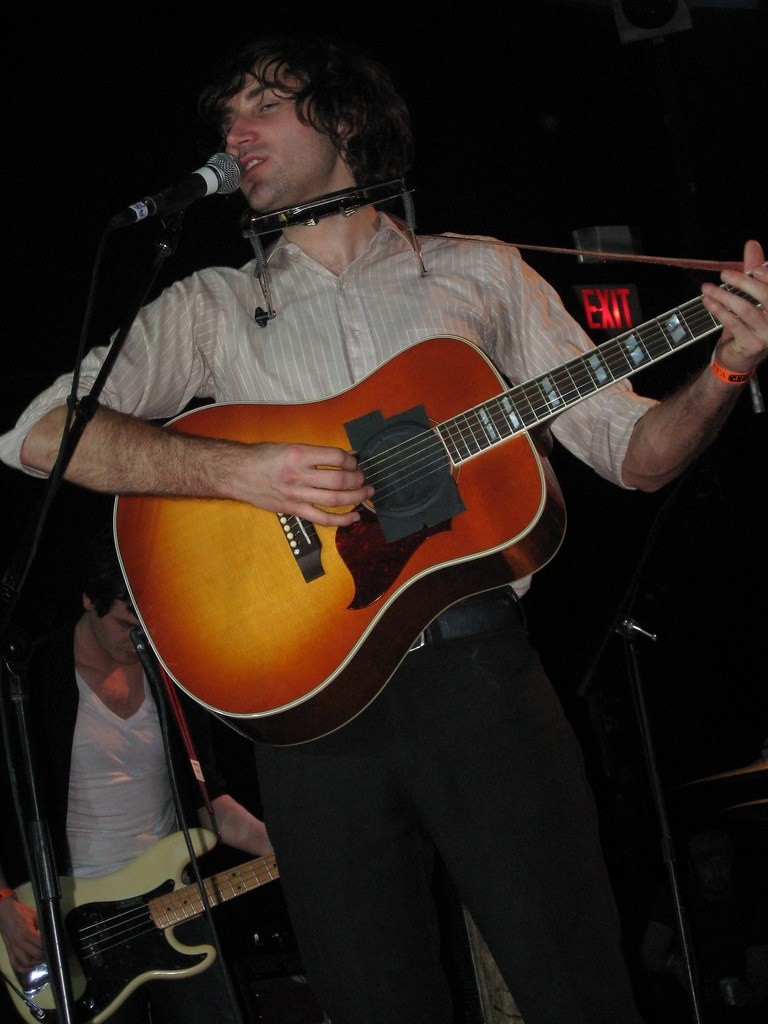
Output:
(0, 28), (768, 1024)
(0, 562), (273, 1024)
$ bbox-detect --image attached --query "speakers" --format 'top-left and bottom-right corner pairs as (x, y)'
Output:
(672, 760), (768, 1024)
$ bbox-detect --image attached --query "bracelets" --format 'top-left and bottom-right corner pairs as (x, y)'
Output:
(710, 360), (757, 385)
(0, 887), (19, 905)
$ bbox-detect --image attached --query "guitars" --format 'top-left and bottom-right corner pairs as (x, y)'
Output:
(0, 825), (282, 1024)
(108, 259), (768, 752)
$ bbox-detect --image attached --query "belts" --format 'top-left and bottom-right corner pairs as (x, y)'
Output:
(425, 598), (521, 645)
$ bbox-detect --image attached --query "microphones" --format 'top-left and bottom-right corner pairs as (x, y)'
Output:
(749, 375), (765, 414)
(107, 152), (245, 230)
